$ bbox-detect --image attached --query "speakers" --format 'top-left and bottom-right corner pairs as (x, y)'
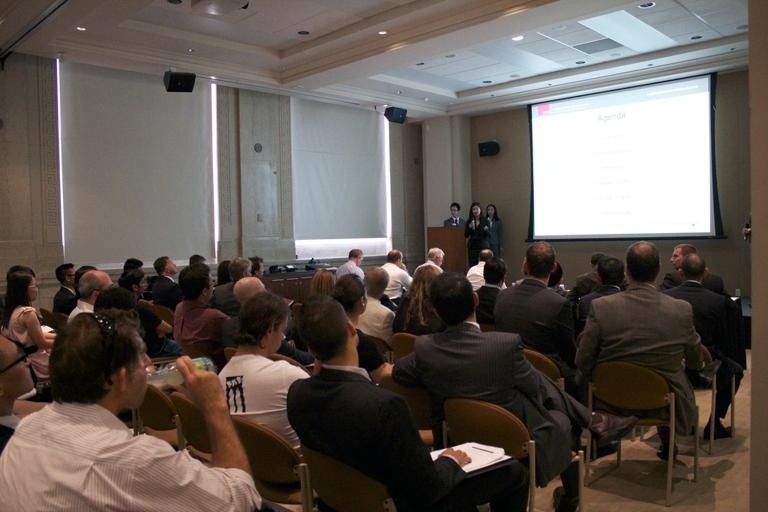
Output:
(163, 71), (197, 92)
(384, 106), (408, 125)
(478, 141), (500, 157)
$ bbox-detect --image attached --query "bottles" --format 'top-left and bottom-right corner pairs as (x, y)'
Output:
(147, 357), (217, 389)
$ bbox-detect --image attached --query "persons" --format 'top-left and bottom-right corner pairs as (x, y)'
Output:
(1, 234), (748, 511)
(465, 202), (490, 270)
(443, 200), (464, 226)
(484, 204), (503, 258)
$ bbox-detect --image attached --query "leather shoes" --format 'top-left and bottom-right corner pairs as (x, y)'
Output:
(552, 486), (578, 511)
(704, 424), (731, 440)
(590, 412), (638, 447)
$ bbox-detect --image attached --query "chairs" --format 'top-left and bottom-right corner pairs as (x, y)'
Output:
(299, 444), (394, 511)
(440, 396), (538, 511)
(1, 268), (740, 452)
(225, 414), (315, 510)
(582, 360), (699, 506)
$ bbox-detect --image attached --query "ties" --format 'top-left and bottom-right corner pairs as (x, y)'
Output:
(455, 219), (459, 225)
